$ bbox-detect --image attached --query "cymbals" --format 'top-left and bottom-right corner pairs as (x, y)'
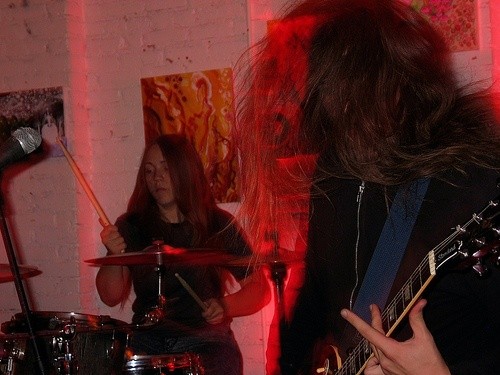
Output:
(0, 264), (42, 284)
(227, 248), (309, 266)
(84, 248), (225, 265)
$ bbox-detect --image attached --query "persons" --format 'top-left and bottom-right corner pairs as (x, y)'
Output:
(272, 0), (500, 375)
(94, 134), (271, 375)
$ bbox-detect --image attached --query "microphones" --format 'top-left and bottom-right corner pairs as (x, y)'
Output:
(0, 127), (41, 167)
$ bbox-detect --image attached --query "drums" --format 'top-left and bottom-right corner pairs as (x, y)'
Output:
(15, 311), (128, 375)
(124, 353), (203, 375)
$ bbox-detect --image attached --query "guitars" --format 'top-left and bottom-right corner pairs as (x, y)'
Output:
(315, 200), (500, 375)
